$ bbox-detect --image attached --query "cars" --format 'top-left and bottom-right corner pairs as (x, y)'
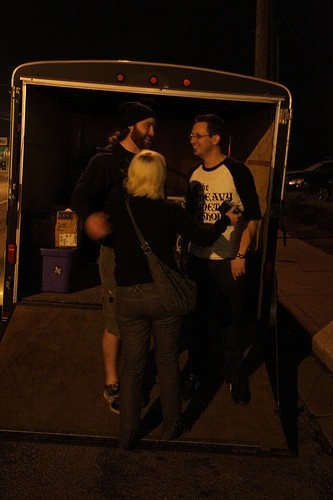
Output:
(284, 159), (333, 203)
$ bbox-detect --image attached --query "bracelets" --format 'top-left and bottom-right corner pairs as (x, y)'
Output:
(236, 252), (247, 260)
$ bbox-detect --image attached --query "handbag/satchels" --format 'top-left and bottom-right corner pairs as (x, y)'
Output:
(124, 198), (197, 314)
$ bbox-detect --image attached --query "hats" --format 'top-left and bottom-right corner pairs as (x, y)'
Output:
(119, 102), (156, 129)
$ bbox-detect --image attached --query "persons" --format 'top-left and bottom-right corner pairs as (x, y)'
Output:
(71, 101), (242, 454)
(181, 115), (263, 405)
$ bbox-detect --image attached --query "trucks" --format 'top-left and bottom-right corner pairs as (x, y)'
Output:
(0, 59), (294, 457)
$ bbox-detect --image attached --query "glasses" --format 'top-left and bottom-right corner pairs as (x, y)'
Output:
(188, 132), (210, 139)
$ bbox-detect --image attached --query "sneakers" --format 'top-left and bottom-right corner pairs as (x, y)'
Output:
(104, 380), (120, 414)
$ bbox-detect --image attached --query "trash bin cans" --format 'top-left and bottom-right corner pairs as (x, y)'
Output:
(40, 247), (76, 294)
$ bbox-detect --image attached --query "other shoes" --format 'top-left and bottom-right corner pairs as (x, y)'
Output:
(161, 426), (183, 440)
(180, 359), (200, 400)
(120, 431), (145, 450)
(226, 370), (251, 404)
